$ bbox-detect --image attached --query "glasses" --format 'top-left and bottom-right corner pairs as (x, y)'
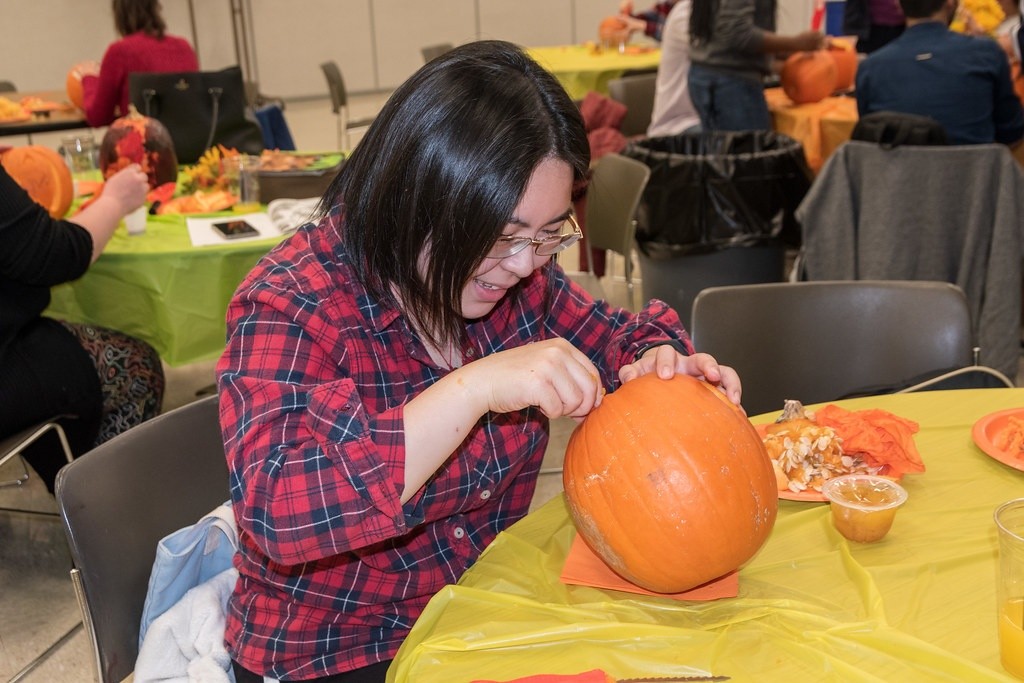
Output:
(479, 212), (588, 259)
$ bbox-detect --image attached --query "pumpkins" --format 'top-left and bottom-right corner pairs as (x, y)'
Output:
(97, 105), (178, 193)
(597, 16), (632, 44)
(562, 371), (778, 595)
(0, 145), (74, 219)
(782, 43), (857, 103)
(65, 64), (86, 108)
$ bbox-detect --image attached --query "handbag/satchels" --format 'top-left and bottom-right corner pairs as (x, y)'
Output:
(129, 67), (264, 167)
(140, 500), (237, 655)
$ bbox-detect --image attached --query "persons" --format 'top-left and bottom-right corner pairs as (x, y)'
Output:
(648, 0), (703, 137)
(0, 159), (166, 498)
(844, 0), (1024, 146)
(619, 0), (678, 41)
(214, 39), (749, 683)
(82, 0), (202, 125)
(686, 0), (826, 130)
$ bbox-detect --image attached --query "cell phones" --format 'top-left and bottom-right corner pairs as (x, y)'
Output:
(212, 220), (258, 238)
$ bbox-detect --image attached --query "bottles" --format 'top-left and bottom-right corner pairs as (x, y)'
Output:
(226, 156), (259, 216)
(60, 136), (101, 199)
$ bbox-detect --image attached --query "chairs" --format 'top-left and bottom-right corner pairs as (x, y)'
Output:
(795, 142), (1024, 388)
(54, 394), (237, 683)
(319, 58), (374, 152)
(0, 83), (32, 145)
(695, 277), (972, 413)
(611, 77), (655, 137)
(584, 151), (651, 306)
(0, 421), (122, 683)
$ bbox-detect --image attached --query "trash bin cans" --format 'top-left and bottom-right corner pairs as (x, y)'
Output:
(621, 129), (804, 336)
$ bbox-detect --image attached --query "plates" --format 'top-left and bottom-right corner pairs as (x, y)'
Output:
(752, 421), (905, 503)
(971, 405), (1024, 472)
(0, 113), (32, 125)
(29, 102), (57, 113)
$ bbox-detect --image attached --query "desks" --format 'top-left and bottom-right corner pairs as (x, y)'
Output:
(379, 386), (1024, 683)
(0, 92), (119, 133)
(767, 87), (855, 169)
(43, 151), (351, 368)
(525, 45), (661, 99)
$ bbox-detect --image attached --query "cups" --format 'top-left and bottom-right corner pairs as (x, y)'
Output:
(994, 499), (1024, 680)
(822, 473), (912, 545)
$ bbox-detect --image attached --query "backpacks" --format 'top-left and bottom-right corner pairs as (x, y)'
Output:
(850, 106), (958, 150)
